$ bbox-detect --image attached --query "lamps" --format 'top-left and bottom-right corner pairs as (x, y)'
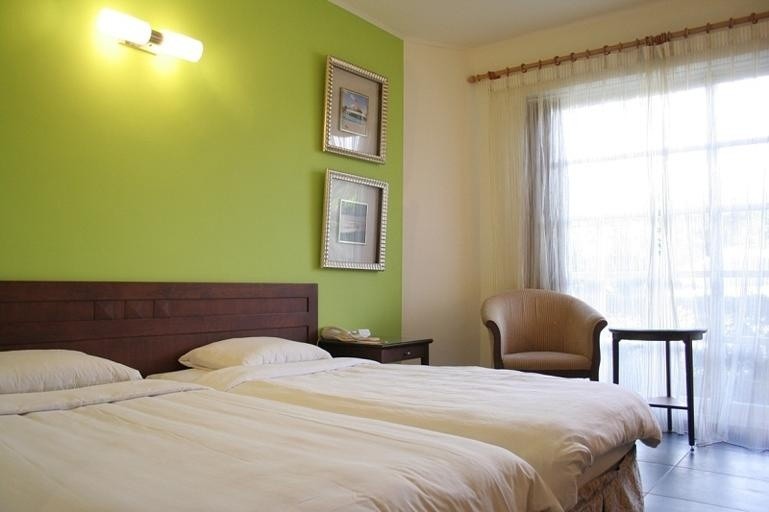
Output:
(99, 9), (205, 65)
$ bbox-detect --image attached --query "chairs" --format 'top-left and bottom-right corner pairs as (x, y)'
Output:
(480, 286), (609, 381)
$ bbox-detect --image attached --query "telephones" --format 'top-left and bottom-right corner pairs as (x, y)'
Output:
(321, 326), (357, 341)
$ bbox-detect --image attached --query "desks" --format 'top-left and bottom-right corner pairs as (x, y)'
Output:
(610, 324), (709, 454)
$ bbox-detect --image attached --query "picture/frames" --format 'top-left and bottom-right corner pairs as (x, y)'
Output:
(321, 53), (391, 165)
(320, 168), (389, 272)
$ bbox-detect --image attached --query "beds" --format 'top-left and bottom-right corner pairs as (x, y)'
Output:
(0, 280), (564, 512)
(146, 282), (661, 512)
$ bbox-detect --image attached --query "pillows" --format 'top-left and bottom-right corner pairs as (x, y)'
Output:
(0, 347), (146, 395)
(176, 334), (332, 373)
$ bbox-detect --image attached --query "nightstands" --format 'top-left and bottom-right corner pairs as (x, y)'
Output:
(320, 336), (433, 369)
